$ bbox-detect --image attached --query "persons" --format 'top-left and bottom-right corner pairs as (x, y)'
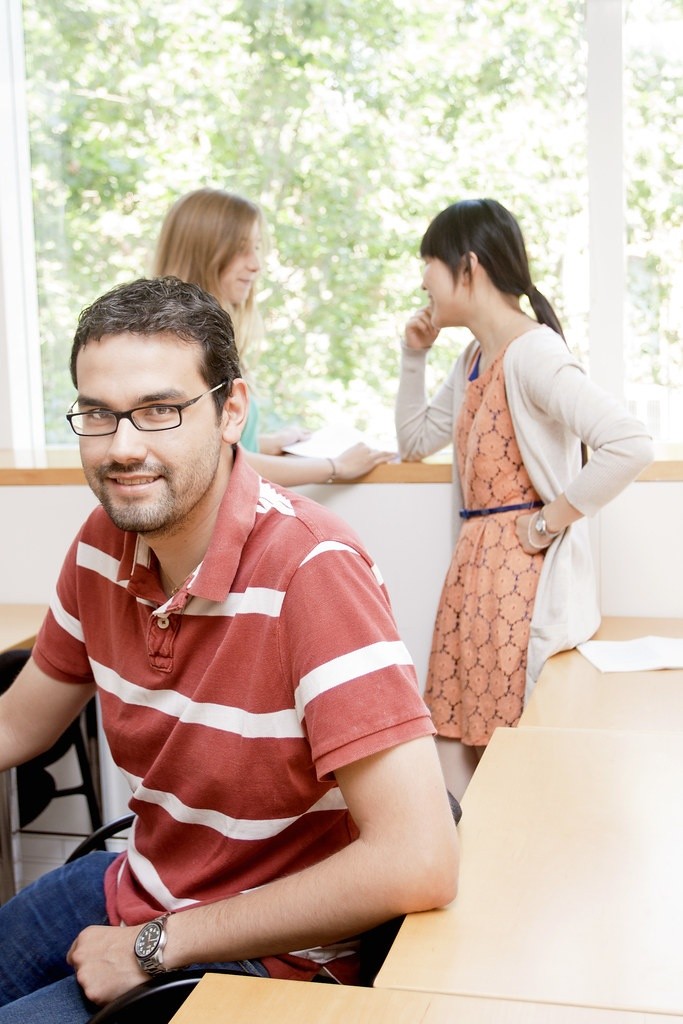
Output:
(396, 197), (653, 806)
(0, 277), (457, 1024)
(150, 185), (401, 489)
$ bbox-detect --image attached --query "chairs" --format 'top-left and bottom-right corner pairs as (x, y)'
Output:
(63, 788), (463, 1024)
(0, 648), (107, 852)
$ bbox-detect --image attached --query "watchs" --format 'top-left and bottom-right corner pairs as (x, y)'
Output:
(132, 910), (179, 976)
(534, 501), (566, 538)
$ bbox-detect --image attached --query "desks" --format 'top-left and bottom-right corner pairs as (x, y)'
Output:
(372, 727), (683, 1016)
(0, 603), (102, 908)
(517, 615), (683, 727)
(168, 972), (682, 1024)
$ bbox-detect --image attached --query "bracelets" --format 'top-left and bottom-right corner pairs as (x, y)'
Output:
(525, 510), (554, 549)
(321, 455), (337, 486)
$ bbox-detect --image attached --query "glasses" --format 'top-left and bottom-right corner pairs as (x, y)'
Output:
(68, 385), (225, 436)
